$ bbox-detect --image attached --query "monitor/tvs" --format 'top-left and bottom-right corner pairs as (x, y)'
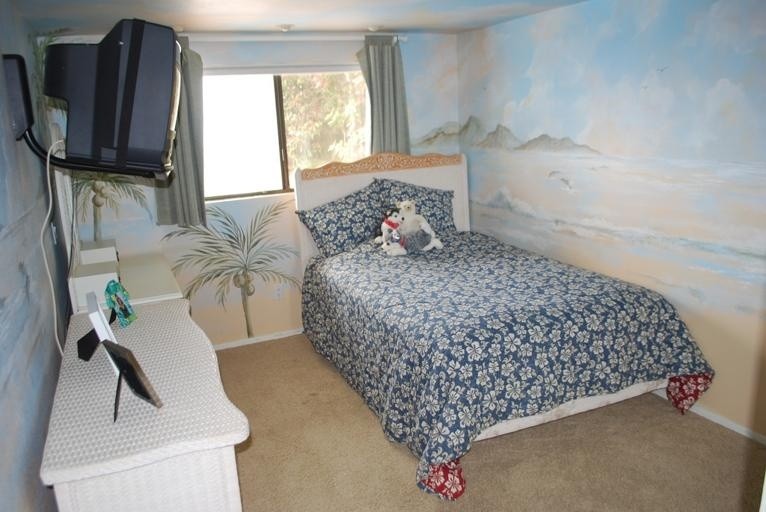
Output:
(42, 18), (182, 170)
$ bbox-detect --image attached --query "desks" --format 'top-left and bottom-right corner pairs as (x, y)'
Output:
(37, 296), (252, 511)
(67, 237), (182, 312)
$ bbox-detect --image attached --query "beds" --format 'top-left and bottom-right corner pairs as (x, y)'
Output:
(289, 151), (717, 504)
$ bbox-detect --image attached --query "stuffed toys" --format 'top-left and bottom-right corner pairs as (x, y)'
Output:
(374, 200), (443, 258)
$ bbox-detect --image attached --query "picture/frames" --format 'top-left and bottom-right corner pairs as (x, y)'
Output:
(99, 279), (141, 326)
(96, 338), (165, 425)
(77, 292), (119, 361)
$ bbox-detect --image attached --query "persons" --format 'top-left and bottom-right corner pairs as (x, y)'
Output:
(115, 294), (130, 319)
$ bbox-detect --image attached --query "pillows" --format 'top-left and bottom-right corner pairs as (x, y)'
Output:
(294, 173), (391, 260)
(371, 176), (458, 241)
